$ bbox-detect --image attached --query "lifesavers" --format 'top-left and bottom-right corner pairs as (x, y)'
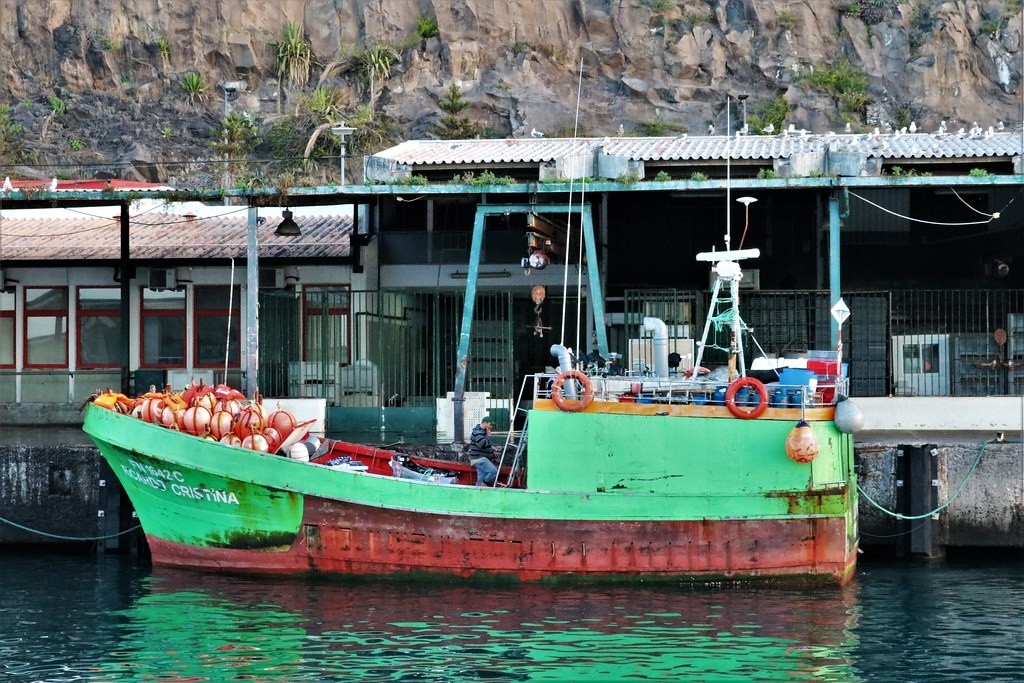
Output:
(724, 376), (770, 420)
(550, 369), (594, 412)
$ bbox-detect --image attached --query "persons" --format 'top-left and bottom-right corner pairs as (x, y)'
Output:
(467, 416), (498, 486)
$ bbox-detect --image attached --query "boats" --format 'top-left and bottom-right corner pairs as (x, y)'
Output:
(82, 93), (861, 591)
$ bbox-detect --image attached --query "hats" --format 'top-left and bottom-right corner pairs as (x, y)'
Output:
(482, 416), (497, 425)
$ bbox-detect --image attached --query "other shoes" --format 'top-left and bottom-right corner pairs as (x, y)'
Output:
(482, 479), (493, 487)
